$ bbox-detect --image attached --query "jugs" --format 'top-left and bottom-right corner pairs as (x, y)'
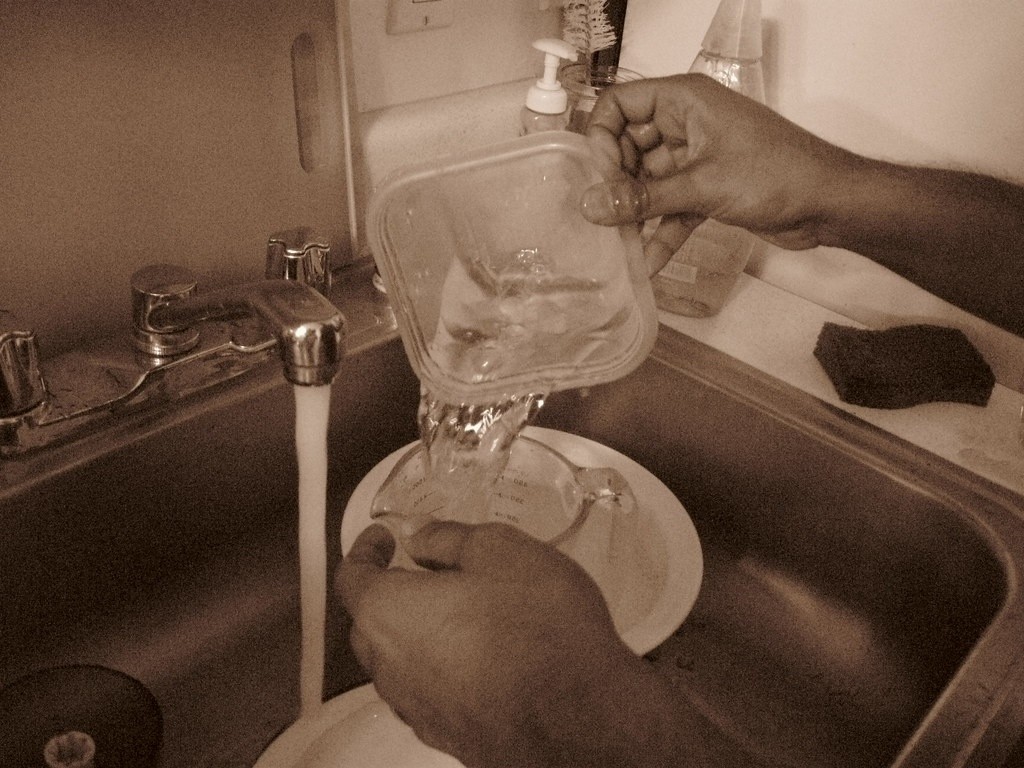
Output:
(370, 433), (639, 569)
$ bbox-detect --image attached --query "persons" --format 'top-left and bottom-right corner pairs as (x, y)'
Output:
(328, 70), (1024, 768)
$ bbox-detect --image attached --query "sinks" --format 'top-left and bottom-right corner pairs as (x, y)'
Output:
(0, 318), (1024, 768)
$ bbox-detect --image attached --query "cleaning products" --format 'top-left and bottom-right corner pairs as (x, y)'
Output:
(526, 36), (581, 136)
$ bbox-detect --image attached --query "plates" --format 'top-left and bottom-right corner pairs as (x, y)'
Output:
(341, 428), (703, 656)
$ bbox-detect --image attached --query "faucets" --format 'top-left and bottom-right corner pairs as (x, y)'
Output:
(127, 224), (348, 389)
